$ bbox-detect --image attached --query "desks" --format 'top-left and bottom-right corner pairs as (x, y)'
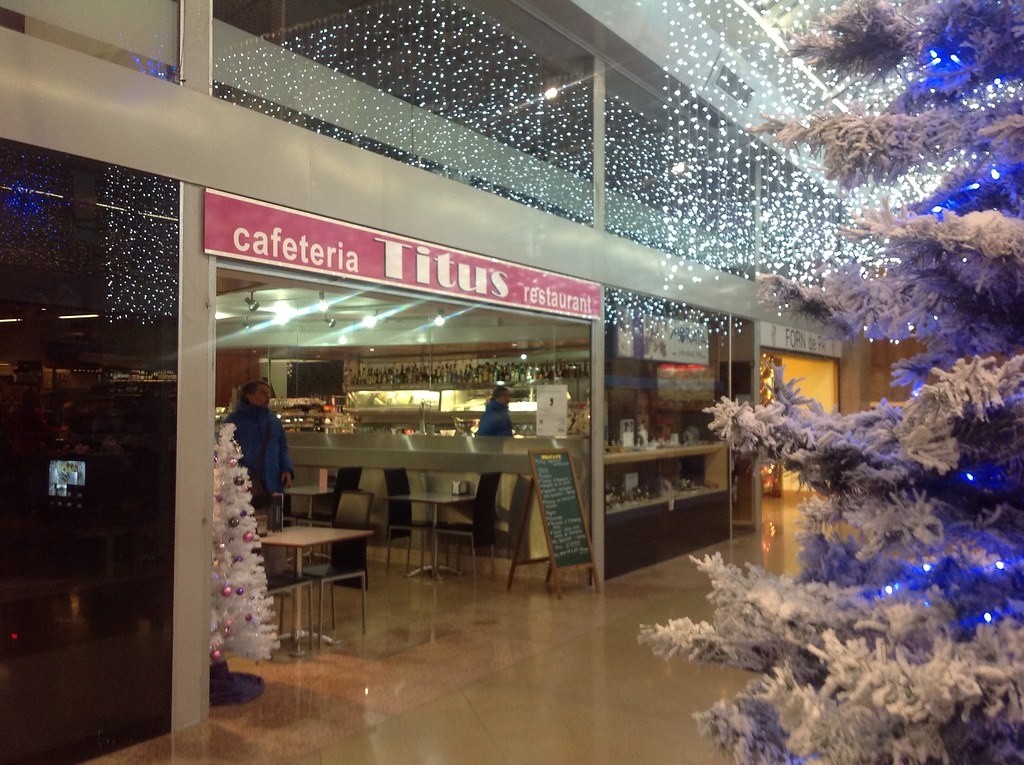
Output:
(261, 526), (376, 658)
(284, 485), (334, 565)
(384, 491), (476, 591)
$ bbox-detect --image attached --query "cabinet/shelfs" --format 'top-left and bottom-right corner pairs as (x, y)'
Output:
(342, 377), (554, 424)
(604, 440), (728, 516)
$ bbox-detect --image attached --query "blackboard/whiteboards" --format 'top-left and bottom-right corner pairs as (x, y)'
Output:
(528, 448), (595, 573)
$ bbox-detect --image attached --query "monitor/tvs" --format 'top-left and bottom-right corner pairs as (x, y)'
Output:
(49, 461), (85, 498)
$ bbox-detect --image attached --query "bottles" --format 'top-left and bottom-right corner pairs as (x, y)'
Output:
(73, 368), (177, 381)
(345, 361), (587, 385)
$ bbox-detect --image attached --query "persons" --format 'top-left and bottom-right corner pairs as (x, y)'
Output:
(224, 381), (295, 510)
(472, 386), (514, 548)
(0, 363), (177, 462)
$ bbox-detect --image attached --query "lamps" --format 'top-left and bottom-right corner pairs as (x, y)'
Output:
(245, 292), (260, 312)
(323, 315), (337, 328)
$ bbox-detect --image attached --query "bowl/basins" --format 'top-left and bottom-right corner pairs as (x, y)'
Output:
(454, 419), (481, 438)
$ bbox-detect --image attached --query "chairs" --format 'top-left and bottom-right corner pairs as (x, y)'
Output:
(302, 489), (375, 649)
(432, 472), (502, 585)
(251, 527), (313, 665)
(284, 494), (322, 556)
(383, 465), (449, 578)
(302, 467), (364, 564)
